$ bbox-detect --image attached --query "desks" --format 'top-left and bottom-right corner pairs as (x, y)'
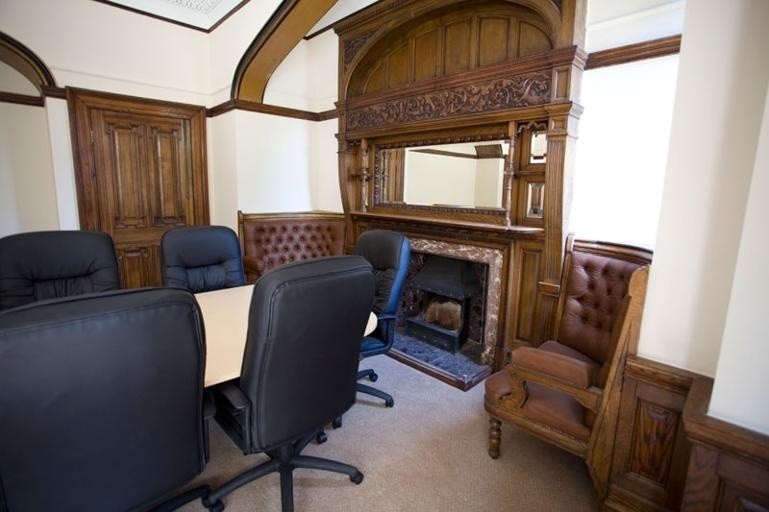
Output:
(194, 285), (379, 390)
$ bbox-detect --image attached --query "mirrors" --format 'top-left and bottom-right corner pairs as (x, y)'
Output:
(366, 121), (517, 228)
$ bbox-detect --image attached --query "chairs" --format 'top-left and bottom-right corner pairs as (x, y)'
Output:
(352, 230), (410, 407)
(208, 255), (375, 512)
(1, 231), (122, 311)
(483, 239), (653, 459)
(159, 225), (246, 295)
(239, 211), (346, 283)
(0, 287), (212, 512)
